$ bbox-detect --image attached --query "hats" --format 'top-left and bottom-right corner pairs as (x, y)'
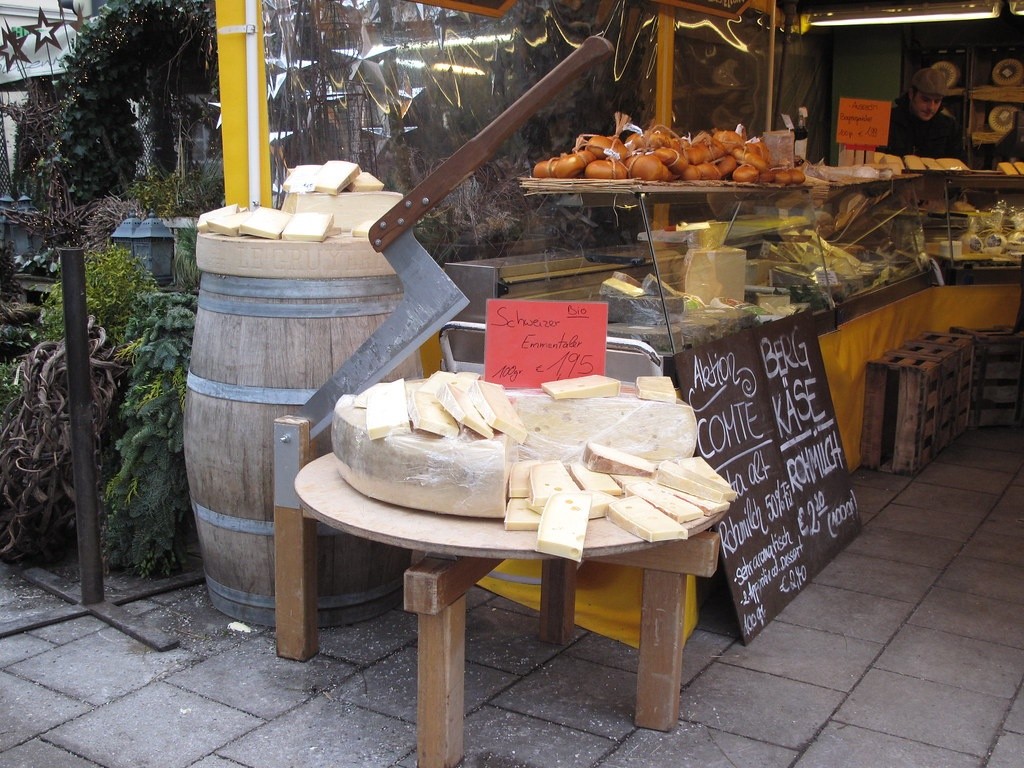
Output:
(911, 68), (949, 97)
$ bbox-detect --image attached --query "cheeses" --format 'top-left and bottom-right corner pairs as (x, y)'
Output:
(197, 157), (405, 241)
(600, 245), (747, 305)
(329, 371), (740, 562)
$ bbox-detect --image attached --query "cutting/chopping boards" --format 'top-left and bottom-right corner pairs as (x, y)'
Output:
(291, 445), (730, 560)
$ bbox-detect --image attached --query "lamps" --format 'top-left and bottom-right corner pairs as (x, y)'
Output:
(795, 0), (1001, 31)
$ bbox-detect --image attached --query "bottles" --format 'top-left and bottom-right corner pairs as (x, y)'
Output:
(793, 107), (810, 161)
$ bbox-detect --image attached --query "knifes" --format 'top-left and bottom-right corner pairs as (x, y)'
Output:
(289, 31), (614, 445)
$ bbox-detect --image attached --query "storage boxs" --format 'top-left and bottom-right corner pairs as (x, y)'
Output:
(954, 326), (1024, 430)
(857, 330), (976, 481)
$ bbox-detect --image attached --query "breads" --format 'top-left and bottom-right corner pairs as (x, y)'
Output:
(873, 151), (1024, 262)
(531, 129), (806, 185)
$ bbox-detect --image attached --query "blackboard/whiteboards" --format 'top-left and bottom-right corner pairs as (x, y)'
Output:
(673, 310), (863, 649)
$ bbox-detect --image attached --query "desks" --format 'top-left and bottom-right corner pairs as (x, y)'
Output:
(270, 414), (739, 767)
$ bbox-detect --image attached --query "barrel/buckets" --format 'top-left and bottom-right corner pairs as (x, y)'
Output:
(178, 268), (426, 631)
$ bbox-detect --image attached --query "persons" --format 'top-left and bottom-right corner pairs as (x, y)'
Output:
(872, 67), (966, 160)
